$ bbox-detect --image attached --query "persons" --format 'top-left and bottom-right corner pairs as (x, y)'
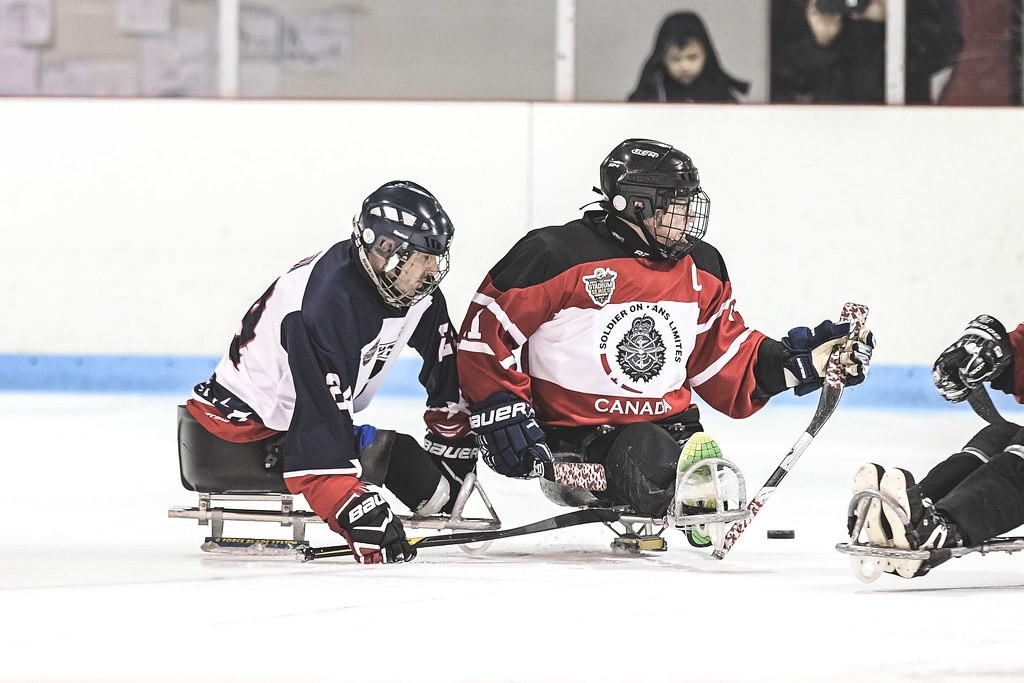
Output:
(834, 314), (1024, 579)
(453, 138), (879, 551)
(177, 178), (482, 563)
(781, 0), (963, 104)
(626, 11), (749, 104)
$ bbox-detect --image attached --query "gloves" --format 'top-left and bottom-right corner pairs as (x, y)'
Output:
(934, 315), (1012, 402)
(780, 320), (873, 396)
(327, 484), (417, 565)
(469, 399), (553, 480)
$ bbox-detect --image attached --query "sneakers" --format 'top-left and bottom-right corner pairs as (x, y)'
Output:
(851, 464), (941, 576)
(675, 430), (726, 547)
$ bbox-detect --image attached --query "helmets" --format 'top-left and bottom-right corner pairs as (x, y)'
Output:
(601, 137), (710, 264)
(353, 178), (450, 306)
